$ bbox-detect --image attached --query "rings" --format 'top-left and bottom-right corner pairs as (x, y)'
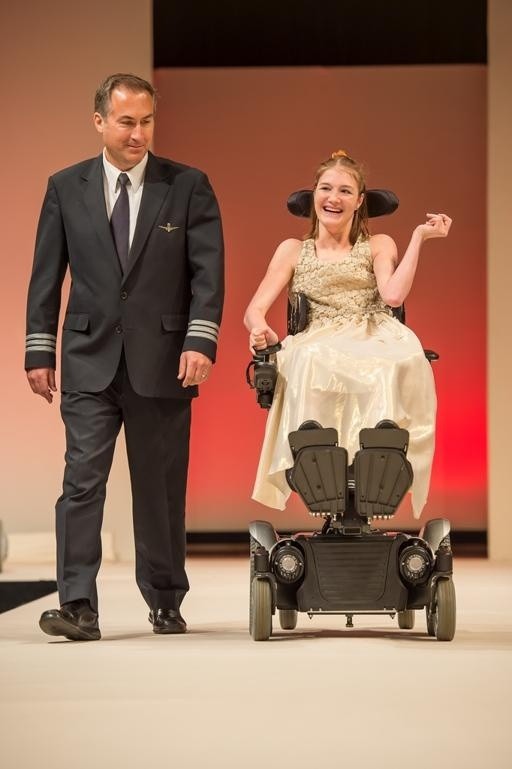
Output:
(201, 373), (207, 378)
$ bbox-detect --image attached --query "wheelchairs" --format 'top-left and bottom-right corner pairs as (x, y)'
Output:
(245, 186), (458, 641)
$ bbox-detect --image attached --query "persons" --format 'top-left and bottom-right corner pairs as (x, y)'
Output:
(241, 148), (453, 518)
(23, 71), (226, 641)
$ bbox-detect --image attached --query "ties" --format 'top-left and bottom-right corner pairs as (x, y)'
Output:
(109, 173), (130, 275)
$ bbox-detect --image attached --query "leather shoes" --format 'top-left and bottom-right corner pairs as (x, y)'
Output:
(39, 599), (101, 640)
(149, 608), (187, 633)
